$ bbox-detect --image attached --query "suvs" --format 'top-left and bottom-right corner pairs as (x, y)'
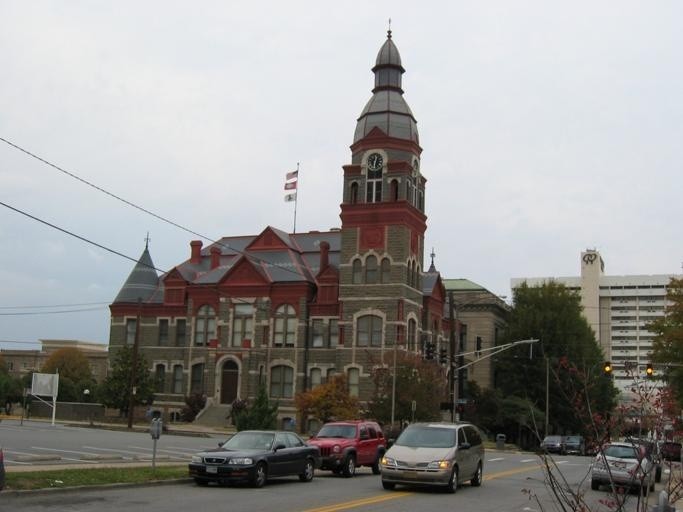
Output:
(305, 421), (388, 480)
(591, 440), (655, 496)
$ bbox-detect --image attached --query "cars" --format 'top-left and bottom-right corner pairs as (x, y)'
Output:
(188, 431), (324, 489)
(541, 430), (682, 484)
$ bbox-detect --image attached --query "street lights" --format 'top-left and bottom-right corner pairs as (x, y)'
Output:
(476, 336), (482, 355)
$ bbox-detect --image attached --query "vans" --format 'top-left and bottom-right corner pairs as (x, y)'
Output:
(380, 421), (485, 493)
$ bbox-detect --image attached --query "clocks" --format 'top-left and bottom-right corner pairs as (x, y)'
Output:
(364, 152), (383, 171)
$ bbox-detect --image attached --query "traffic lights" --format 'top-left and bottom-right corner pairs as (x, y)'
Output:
(457, 404), (463, 414)
(647, 362), (653, 379)
(427, 344), (435, 359)
(441, 348), (447, 364)
(605, 362), (611, 376)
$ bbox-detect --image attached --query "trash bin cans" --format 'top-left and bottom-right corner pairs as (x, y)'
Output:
(282, 416), (295, 432)
(145, 410), (161, 422)
(497, 434), (505, 450)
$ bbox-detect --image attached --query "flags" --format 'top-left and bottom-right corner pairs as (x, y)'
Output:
(285, 193), (296, 202)
(285, 181), (296, 190)
(287, 171), (298, 180)
